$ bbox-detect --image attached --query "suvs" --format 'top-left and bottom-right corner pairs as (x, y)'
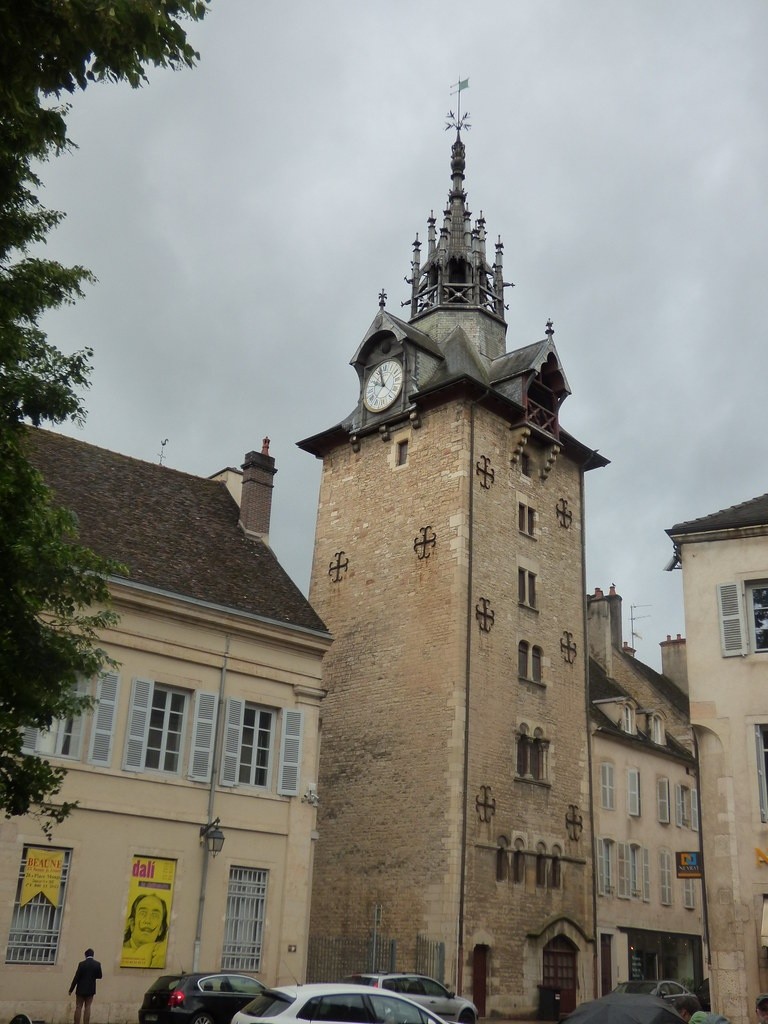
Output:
(341, 972), (479, 1024)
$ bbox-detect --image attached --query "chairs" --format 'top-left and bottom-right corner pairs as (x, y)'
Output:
(328, 998), (349, 1020)
(352, 997), (369, 1021)
(203, 982), (213, 992)
(407, 981), (426, 995)
(220, 981), (237, 992)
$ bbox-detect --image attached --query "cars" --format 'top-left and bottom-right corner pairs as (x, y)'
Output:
(137, 970), (270, 1024)
(579, 980), (703, 1021)
(696, 978), (710, 1011)
(231, 982), (449, 1024)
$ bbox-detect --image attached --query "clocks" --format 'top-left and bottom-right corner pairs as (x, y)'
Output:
(362, 358), (402, 413)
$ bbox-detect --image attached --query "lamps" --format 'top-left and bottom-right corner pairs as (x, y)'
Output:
(199, 817), (224, 858)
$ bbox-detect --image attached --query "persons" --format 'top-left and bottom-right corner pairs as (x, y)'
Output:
(754, 992), (768, 1024)
(674, 995), (731, 1024)
(68, 948), (102, 1024)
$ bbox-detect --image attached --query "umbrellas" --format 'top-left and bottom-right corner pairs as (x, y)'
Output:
(560, 992), (685, 1024)
